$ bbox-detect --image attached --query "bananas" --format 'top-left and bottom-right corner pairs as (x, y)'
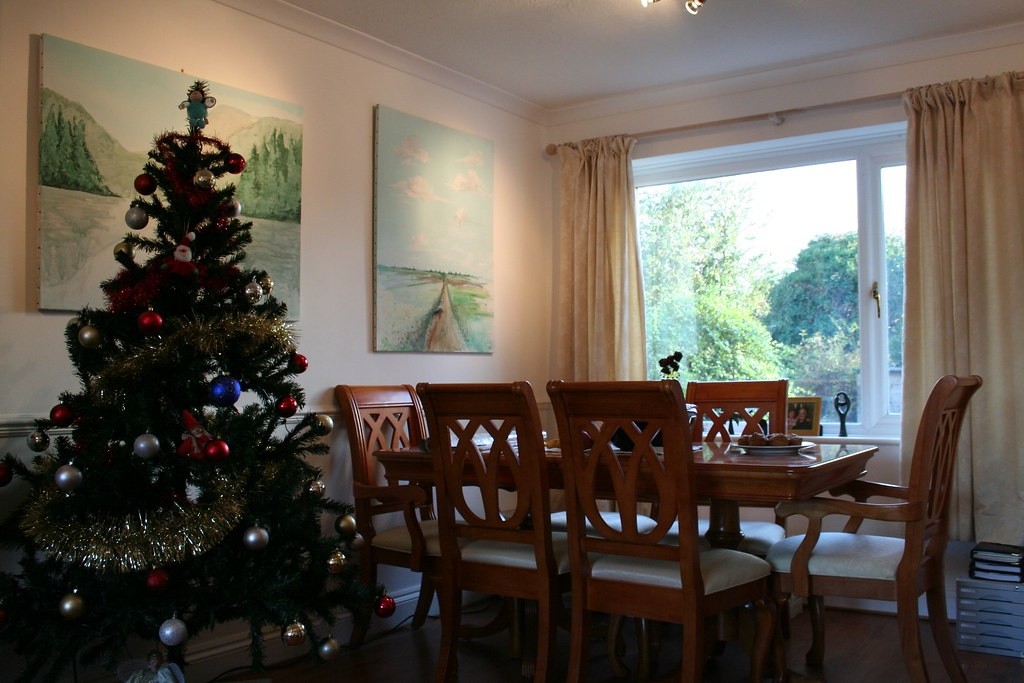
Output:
(545, 439), (561, 448)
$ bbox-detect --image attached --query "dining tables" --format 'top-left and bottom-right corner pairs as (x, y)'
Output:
(370, 440), (883, 683)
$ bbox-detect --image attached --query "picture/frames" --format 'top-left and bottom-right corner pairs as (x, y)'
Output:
(787, 395), (823, 436)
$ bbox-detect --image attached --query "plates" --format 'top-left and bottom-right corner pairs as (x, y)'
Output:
(730, 440), (816, 454)
(454, 430), (547, 449)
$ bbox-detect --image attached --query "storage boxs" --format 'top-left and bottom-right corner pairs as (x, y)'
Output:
(955, 573), (1024, 657)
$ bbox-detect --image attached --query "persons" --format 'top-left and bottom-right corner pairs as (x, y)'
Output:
(787, 408), (815, 429)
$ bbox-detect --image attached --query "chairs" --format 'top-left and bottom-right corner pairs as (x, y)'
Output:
(415, 379), (596, 683)
(650, 379), (791, 683)
(550, 505), (654, 683)
(542, 377), (767, 683)
(768, 371), (984, 683)
(334, 383), (479, 649)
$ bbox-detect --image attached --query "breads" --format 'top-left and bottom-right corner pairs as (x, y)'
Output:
(738, 432), (803, 446)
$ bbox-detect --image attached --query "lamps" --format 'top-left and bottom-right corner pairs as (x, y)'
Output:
(684, 0), (706, 16)
(638, 0), (661, 7)
